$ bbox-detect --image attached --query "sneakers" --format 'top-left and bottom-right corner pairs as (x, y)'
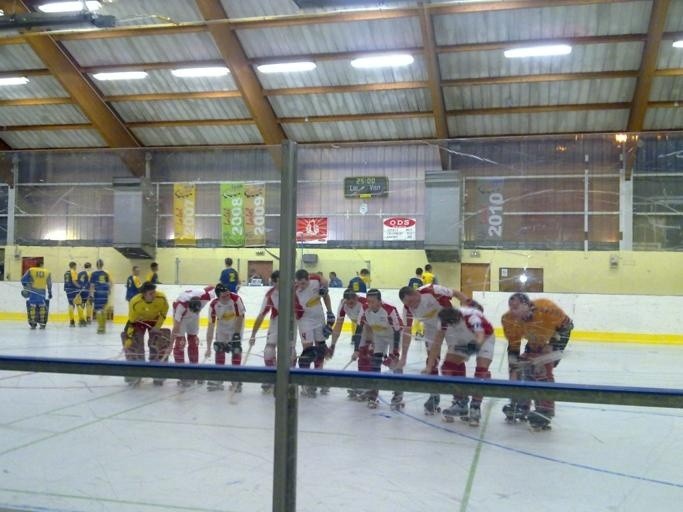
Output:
(503, 402), (555, 423)
(391, 392), (404, 405)
(348, 387), (380, 409)
(28, 317), (108, 334)
(206, 380), (242, 388)
(424, 396), (482, 420)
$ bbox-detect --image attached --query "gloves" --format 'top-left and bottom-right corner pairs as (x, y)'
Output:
(319, 286), (328, 296)
(323, 310), (335, 338)
(214, 334), (242, 355)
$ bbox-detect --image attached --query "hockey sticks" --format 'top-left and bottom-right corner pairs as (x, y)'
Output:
(227, 345), (254, 406)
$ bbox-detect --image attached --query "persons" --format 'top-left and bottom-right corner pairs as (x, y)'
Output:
(293, 265), (574, 434)
(22, 258), (113, 334)
(121, 257), (280, 392)
